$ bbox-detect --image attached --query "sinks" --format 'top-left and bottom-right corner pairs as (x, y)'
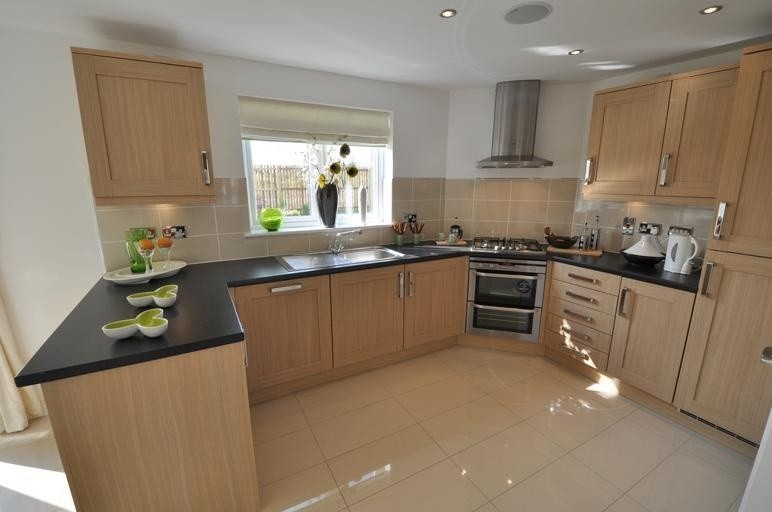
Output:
(272, 251), (343, 271)
(336, 245), (420, 264)
(328, 228), (363, 254)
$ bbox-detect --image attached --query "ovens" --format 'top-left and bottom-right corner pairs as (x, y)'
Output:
(465, 257), (547, 344)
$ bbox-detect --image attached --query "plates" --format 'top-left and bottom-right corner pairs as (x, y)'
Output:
(101, 307), (168, 339)
(127, 284), (179, 307)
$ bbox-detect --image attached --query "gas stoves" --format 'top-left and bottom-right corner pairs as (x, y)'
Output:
(470, 236), (546, 254)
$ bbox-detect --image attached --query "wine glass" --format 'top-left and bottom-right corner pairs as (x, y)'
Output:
(134, 237), (175, 275)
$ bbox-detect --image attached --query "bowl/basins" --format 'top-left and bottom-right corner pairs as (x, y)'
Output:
(621, 248), (666, 265)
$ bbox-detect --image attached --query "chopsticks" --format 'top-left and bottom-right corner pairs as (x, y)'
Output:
(392, 221), (424, 234)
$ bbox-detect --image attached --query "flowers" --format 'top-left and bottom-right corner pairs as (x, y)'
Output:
(296, 132), (358, 190)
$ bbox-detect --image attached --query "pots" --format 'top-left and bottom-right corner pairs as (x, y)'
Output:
(545, 235), (579, 248)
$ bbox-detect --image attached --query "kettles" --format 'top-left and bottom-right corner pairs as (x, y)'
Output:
(663, 224), (701, 275)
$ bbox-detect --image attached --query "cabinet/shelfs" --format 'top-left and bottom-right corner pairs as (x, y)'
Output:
(541, 245), (624, 385)
(601, 257), (698, 418)
(405, 256), (467, 365)
(71, 45), (217, 206)
(234, 256), (329, 407)
(579, 73), (673, 203)
(671, 252), (772, 458)
(655, 62), (740, 211)
(707, 44), (772, 257)
(330, 256), (405, 386)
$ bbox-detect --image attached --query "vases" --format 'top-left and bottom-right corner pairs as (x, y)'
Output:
(260, 208), (284, 230)
(316, 184), (338, 228)
(358, 185), (368, 221)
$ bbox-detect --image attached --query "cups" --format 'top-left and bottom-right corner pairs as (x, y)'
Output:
(396, 234), (405, 245)
(413, 234), (424, 245)
(124, 228), (153, 274)
(437, 225), (463, 242)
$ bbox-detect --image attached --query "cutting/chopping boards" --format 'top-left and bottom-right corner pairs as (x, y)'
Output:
(434, 239), (467, 246)
(547, 244), (603, 256)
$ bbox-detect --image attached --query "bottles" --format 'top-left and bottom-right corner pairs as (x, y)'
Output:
(579, 216), (600, 250)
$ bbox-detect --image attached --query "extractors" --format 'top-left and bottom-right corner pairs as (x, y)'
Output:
(476, 80), (554, 169)
